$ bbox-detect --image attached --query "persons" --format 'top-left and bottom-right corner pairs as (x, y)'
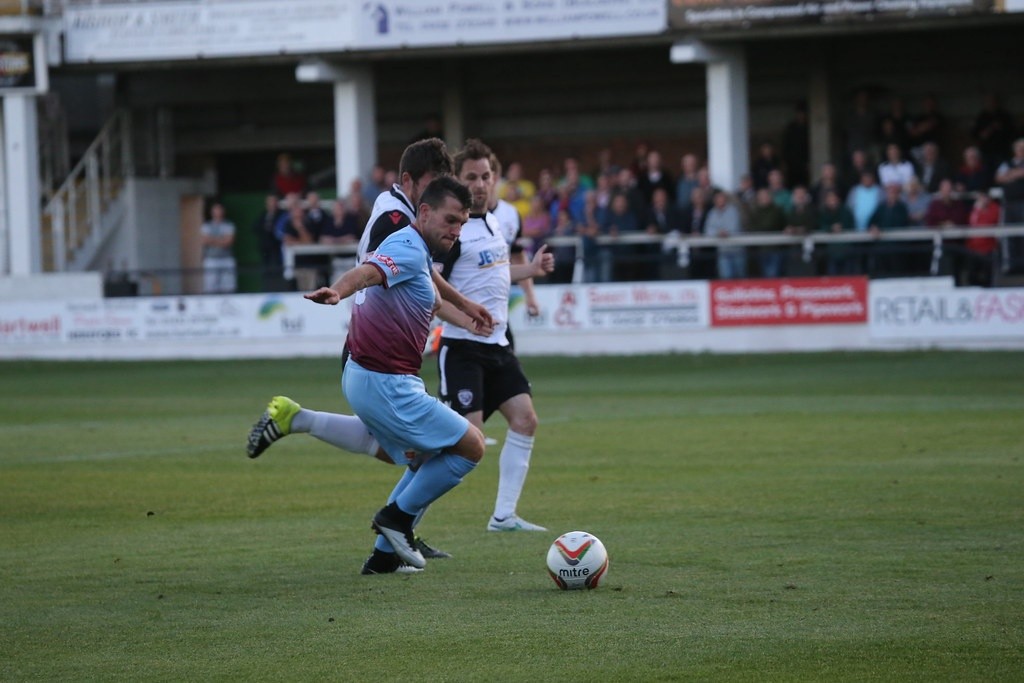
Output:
(496, 88), (1024, 289)
(246, 138), (452, 559)
(198, 201), (237, 293)
(303, 176), (495, 574)
(423, 143), (555, 531)
(255, 152), (400, 291)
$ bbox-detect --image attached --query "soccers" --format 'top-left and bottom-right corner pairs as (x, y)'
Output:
(546, 530), (610, 590)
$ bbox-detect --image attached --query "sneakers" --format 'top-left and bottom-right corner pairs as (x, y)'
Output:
(486, 511), (548, 532)
(246, 395), (301, 458)
(370, 505), (428, 569)
(359, 549), (424, 575)
(412, 536), (454, 559)
